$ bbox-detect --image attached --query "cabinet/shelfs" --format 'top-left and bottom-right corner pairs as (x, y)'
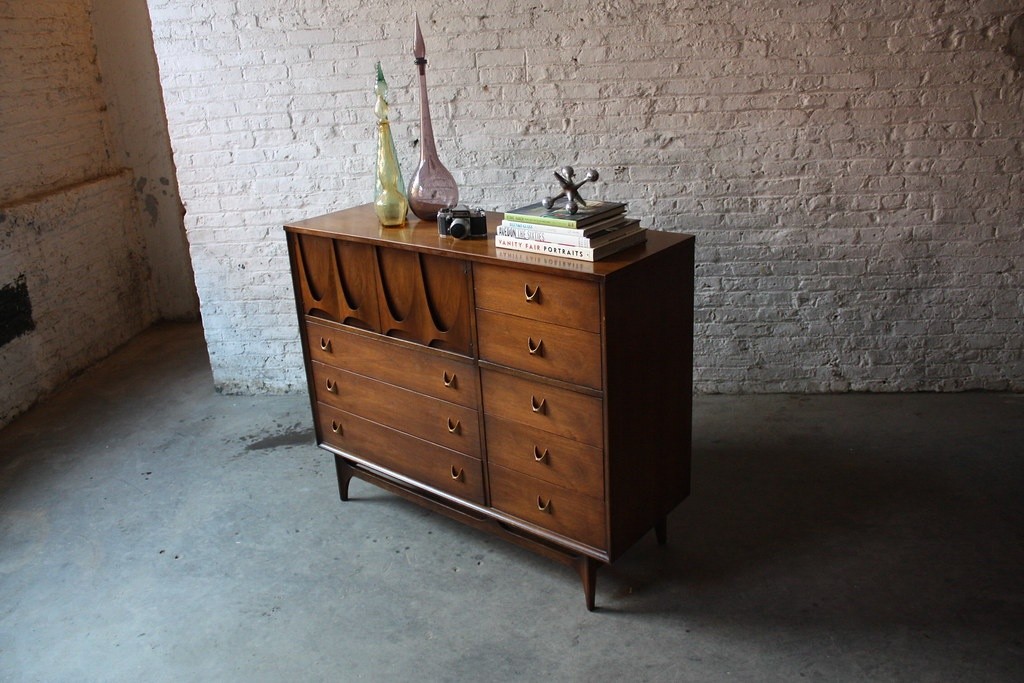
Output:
(282, 202), (695, 612)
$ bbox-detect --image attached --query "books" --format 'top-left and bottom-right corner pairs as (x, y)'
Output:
(496, 197), (647, 262)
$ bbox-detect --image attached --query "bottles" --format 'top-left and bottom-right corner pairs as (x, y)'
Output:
(374, 11), (459, 228)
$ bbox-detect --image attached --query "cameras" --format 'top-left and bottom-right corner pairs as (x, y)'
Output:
(437, 206), (488, 239)
